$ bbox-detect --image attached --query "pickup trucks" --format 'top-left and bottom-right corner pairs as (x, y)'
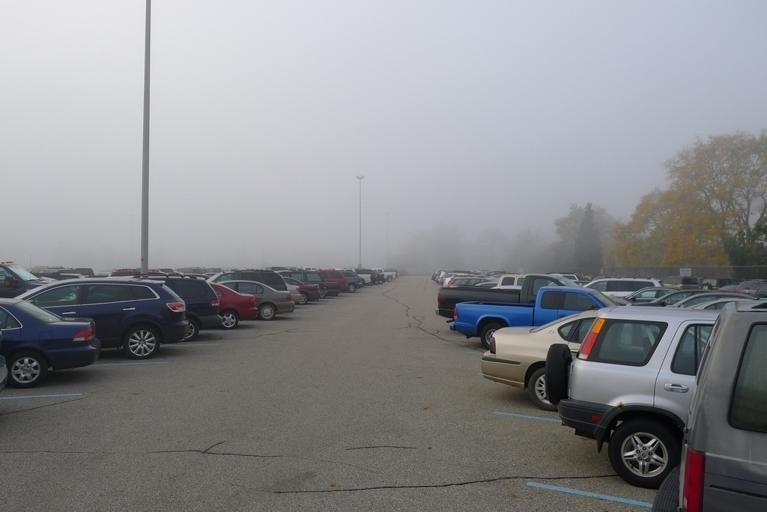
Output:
(434, 274), (581, 324)
(448, 286), (634, 351)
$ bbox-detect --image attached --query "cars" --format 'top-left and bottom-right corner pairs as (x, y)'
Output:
(431, 269), (767, 335)
(0, 357), (5, 386)
(0, 298), (103, 388)
(267, 265), (397, 305)
(29, 265), (221, 282)
(216, 281), (294, 321)
(480, 310), (710, 412)
(206, 280), (258, 330)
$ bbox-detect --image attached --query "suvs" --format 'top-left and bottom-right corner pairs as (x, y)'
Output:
(13, 275), (190, 361)
(206, 269), (288, 293)
(0, 260), (48, 300)
(649, 299), (767, 512)
(544, 306), (721, 491)
(134, 273), (219, 345)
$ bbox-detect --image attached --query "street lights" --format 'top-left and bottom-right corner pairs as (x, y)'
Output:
(355, 176), (364, 268)
